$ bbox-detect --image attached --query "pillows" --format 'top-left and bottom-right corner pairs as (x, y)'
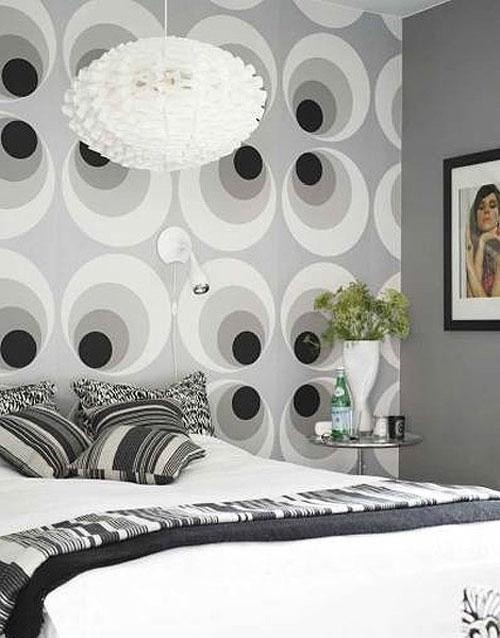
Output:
(84, 397), (182, 433)
(0, 407), (90, 479)
(72, 371), (213, 432)
(66, 423), (208, 486)
(0, 383), (58, 413)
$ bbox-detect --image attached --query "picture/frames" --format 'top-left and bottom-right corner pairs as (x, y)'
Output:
(442, 148), (500, 330)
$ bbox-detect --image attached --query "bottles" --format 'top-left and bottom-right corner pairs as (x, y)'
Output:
(331, 366), (353, 440)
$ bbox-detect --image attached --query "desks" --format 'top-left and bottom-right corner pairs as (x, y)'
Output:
(311, 428), (421, 476)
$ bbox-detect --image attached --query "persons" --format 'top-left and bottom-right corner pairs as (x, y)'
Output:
(464, 184), (500, 297)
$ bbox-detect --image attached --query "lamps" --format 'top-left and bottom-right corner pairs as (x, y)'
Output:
(62, 0), (267, 173)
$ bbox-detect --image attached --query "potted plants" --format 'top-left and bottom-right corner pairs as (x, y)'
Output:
(315, 281), (410, 430)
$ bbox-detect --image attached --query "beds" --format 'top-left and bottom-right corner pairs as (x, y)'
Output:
(0, 434), (500, 638)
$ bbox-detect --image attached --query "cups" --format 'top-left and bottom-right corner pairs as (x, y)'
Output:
(387, 415), (405, 441)
(314, 421), (331, 436)
